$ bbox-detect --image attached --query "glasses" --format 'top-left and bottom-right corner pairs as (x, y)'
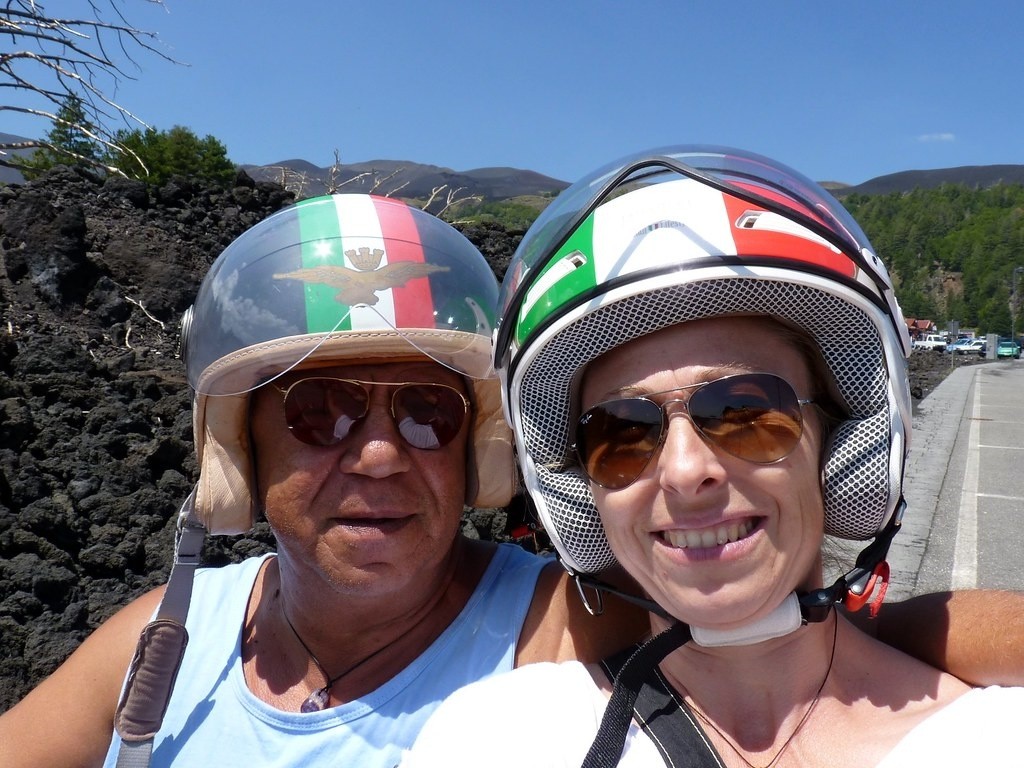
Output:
(268, 377), (473, 452)
(575, 375), (817, 489)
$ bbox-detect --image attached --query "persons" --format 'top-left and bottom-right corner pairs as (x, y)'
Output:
(1, 196), (1023, 768)
(401, 150), (1024, 768)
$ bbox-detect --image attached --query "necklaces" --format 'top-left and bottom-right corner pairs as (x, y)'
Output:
(281, 571), (454, 713)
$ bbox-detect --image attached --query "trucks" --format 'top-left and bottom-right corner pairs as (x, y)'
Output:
(914, 334), (947, 353)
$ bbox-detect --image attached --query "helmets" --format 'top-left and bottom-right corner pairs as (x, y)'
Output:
(494, 143), (915, 768)
(117, 194), (519, 768)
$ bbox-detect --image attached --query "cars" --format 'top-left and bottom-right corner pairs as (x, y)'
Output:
(997, 341), (1021, 359)
(946, 335), (987, 358)
(977, 336), (1011, 357)
